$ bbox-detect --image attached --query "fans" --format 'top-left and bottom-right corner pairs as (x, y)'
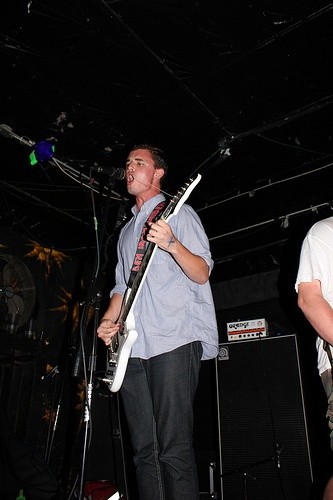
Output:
(0, 253), (38, 337)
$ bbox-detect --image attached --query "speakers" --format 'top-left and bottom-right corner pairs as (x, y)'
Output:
(64, 370), (134, 500)
(214, 333), (315, 500)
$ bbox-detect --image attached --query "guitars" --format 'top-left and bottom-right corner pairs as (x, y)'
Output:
(101, 172), (206, 393)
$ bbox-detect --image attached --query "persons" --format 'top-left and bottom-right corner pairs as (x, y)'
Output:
(98, 144), (219, 500)
(295, 217), (333, 500)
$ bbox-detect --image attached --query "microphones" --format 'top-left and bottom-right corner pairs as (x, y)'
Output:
(92, 166), (125, 180)
(271, 439), (282, 467)
